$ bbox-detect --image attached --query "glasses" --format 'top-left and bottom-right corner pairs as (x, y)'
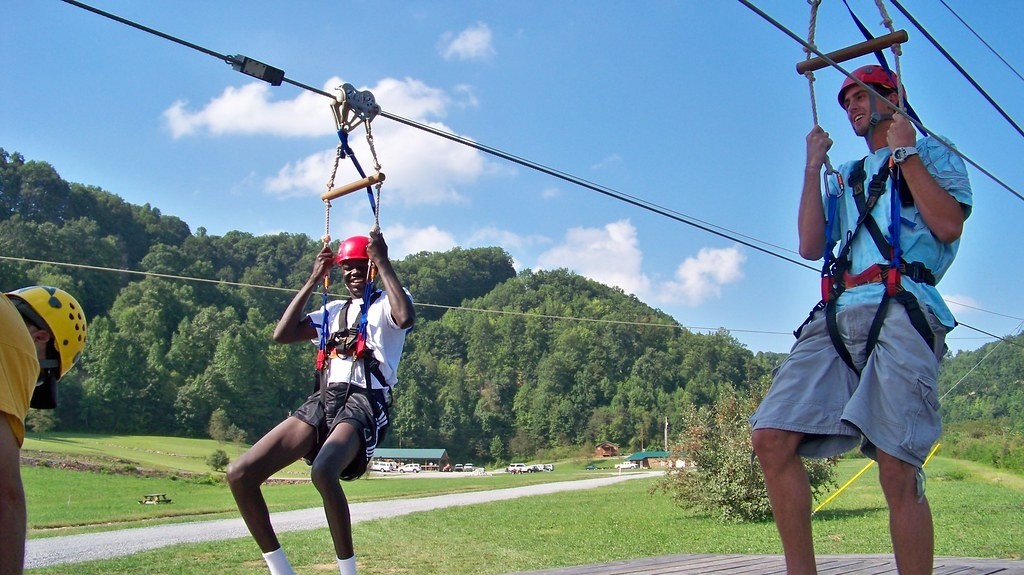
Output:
(35, 368), (50, 387)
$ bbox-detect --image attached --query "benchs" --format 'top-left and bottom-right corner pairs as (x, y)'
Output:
(138, 499), (172, 504)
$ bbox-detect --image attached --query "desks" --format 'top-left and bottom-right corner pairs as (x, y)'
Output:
(143, 493), (167, 504)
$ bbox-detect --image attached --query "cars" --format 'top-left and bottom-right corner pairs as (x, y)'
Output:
(543, 464), (553, 470)
(586, 465), (596, 470)
(455, 464), (462, 471)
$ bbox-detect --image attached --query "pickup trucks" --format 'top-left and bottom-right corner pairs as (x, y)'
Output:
(615, 462), (635, 469)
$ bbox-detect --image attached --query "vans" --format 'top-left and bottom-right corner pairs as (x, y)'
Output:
(370, 462), (392, 472)
(398, 464), (421, 473)
(508, 463), (526, 471)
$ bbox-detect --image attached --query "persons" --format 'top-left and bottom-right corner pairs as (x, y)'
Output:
(226, 231), (417, 574)
(747, 65), (974, 575)
(1, 285), (88, 575)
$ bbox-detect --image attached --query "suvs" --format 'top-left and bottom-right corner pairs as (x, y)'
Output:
(464, 463), (474, 471)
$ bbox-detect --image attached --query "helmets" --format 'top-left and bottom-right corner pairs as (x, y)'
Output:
(838, 64), (908, 113)
(4, 286), (87, 382)
(337, 236), (378, 275)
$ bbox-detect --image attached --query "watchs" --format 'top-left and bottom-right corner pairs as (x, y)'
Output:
(892, 146), (918, 165)
